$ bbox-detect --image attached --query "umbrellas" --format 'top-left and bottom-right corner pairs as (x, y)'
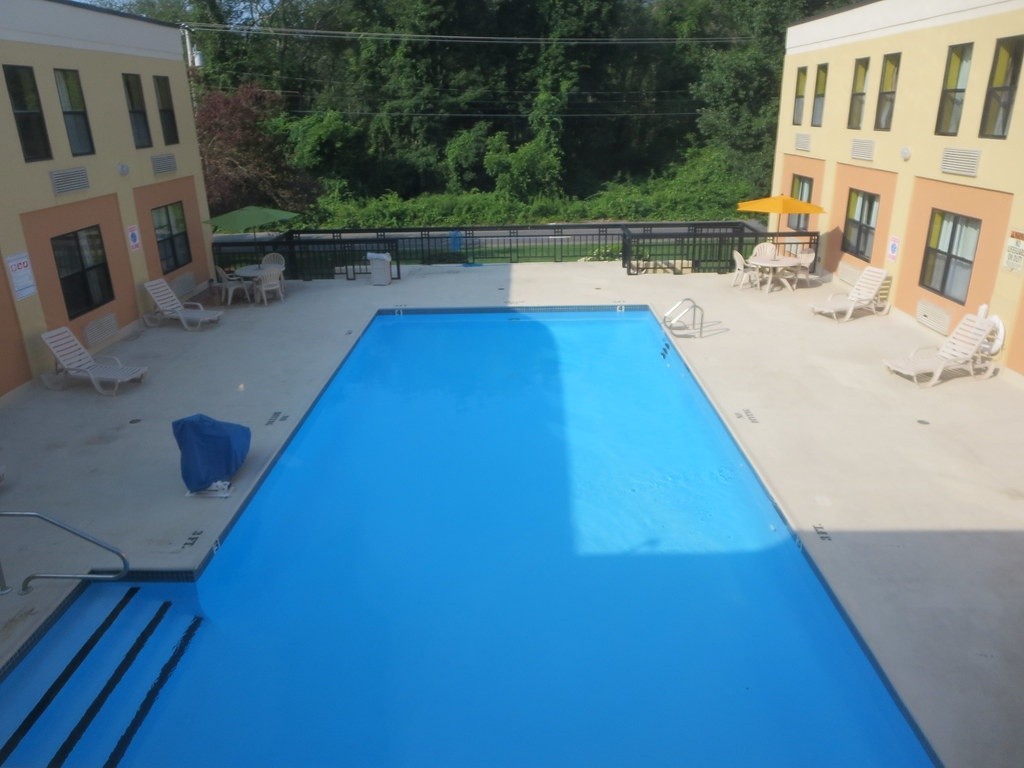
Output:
(203, 206), (300, 270)
(736, 193), (826, 260)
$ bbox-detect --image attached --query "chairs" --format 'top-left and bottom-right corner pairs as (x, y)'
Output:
(808, 266), (891, 323)
(141, 278), (223, 332)
(252, 267), (285, 306)
(731, 249), (761, 291)
(786, 247), (815, 290)
(748, 243), (777, 281)
(261, 252), (286, 293)
(215, 265), (252, 307)
(40, 326), (148, 396)
(884, 313), (1001, 389)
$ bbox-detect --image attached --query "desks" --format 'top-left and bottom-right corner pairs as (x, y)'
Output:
(749, 256), (802, 294)
(234, 263), (286, 304)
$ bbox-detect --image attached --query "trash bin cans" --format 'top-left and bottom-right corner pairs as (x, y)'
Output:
(367, 251), (392, 286)
